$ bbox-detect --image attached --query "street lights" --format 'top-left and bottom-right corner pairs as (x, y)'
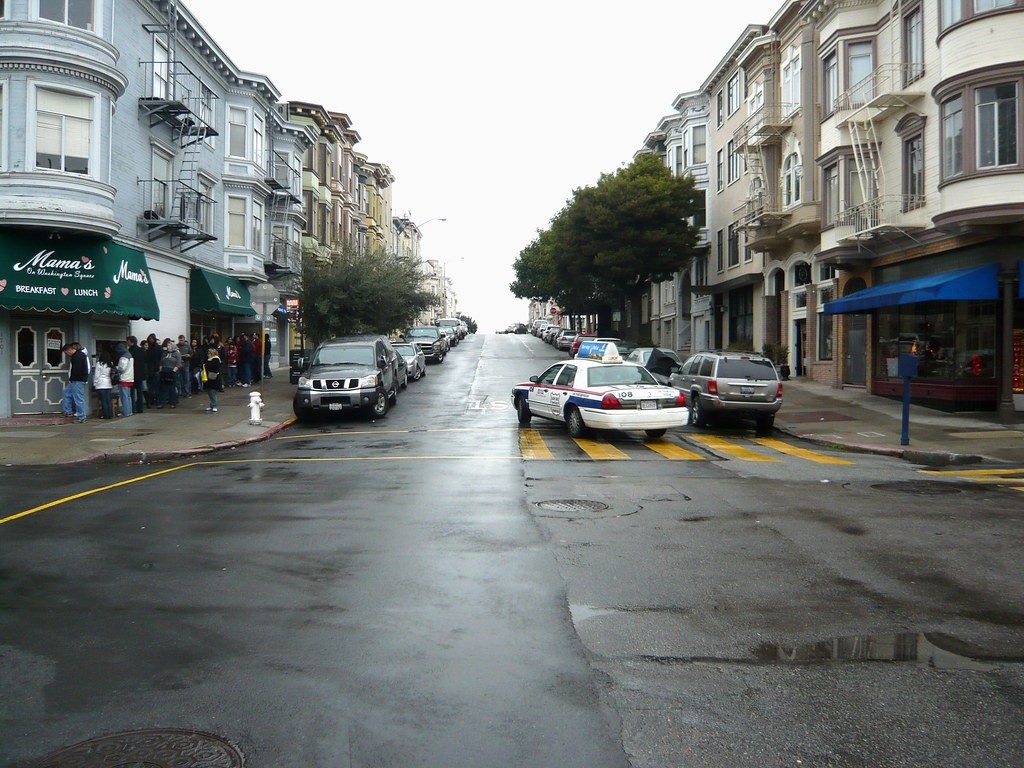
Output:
(415, 217), (447, 326)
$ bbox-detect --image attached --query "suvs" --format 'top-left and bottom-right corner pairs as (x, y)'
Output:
(293, 334), (397, 419)
(400, 326), (446, 363)
(668, 348), (783, 428)
(436, 319), (463, 341)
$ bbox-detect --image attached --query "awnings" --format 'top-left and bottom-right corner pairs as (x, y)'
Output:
(189, 267), (258, 316)
(0, 223), (160, 321)
(1018, 262), (1024, 299)
(822, 262), (999, 314)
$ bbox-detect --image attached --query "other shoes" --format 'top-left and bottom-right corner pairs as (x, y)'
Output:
(212, 408), (217, 411)
(206, 407), (212, 410)
(59, 413), (73, 417)
(156, 404), (164, 409)
(100, 416), (113, 418)
(170, 404), (176, 409)
(117, 413), (132, 417)
(73, 419), (87, 424)
(237, 382), (242, 387)
(242, 383), (251, 388)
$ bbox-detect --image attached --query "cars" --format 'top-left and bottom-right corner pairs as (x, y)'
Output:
(459, 320), (469, 336)
(537, 324), (568, 346)
(531, 320), (549, 335)
(568, 334), (598, 357)
(438, 327), (457, 351)
(595, 338), (639, 360)
(624, 348), (684, 378)
(506, 323), (528, 334)
(556, 331), (579, 350)
(510, 341), (689, 440)
(391, 342), (427, 380)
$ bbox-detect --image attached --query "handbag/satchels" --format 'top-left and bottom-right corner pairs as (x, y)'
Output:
(201, 364), (208, 383)
(208, 372), (218, 380)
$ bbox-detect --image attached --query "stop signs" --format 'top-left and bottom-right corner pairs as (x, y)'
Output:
(550, 308), (556, 314)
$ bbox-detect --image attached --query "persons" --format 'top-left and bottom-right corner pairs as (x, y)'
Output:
(58, 331), (272, 424)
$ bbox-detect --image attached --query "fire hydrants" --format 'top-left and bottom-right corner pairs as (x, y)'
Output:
(247, 392), (265, 425)
